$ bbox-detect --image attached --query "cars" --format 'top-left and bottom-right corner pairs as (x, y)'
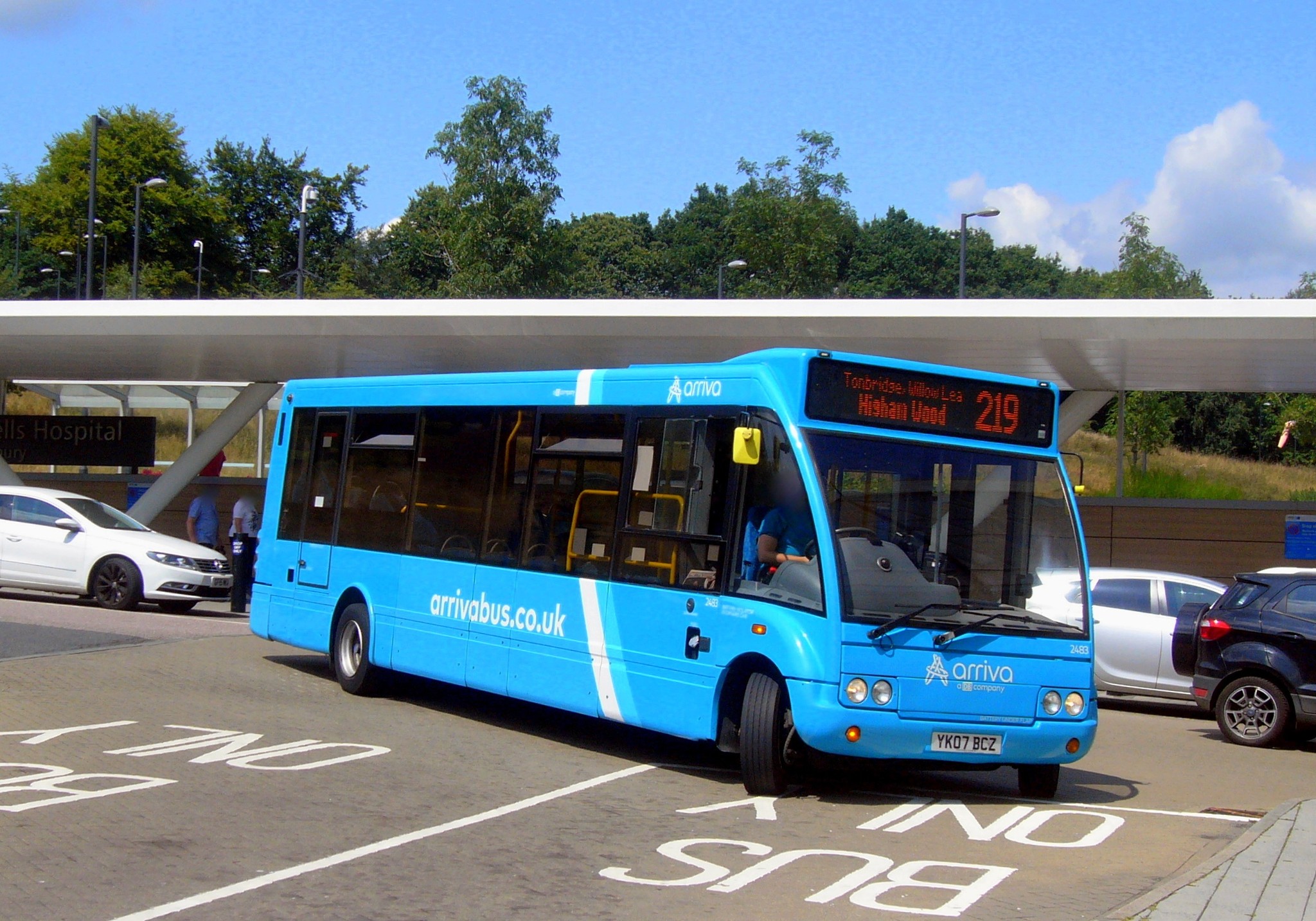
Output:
(0, 482), (235, 612)
(993, 564), (1248, 716)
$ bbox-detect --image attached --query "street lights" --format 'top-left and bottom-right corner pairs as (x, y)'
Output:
(131, 177), (168, 300)
(717, 260), (748, 300)
(59, 251), (81, 300)
(75, 218), (104, 300)
(83, 234), (107, 300)
(0, 209), (20, 295)
(85, 110), (110, 303)
(41, 268), (60, 300)
(958, 207), (1001, 300)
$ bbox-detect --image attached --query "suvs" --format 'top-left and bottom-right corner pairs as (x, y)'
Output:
(1169, 566), (1316, 750)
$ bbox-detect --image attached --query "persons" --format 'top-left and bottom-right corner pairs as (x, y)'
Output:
(759, 481), (852, 584)
(186, 486), (219, 550)
(229, 490), (260, 613)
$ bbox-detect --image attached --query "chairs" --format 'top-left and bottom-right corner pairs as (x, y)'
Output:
(296, 472), (405, 512)
(742, 498), (778, 580)
(415, 518), (560, 569)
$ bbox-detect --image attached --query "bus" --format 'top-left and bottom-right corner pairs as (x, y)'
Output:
(244, 340), (1104, 803)
